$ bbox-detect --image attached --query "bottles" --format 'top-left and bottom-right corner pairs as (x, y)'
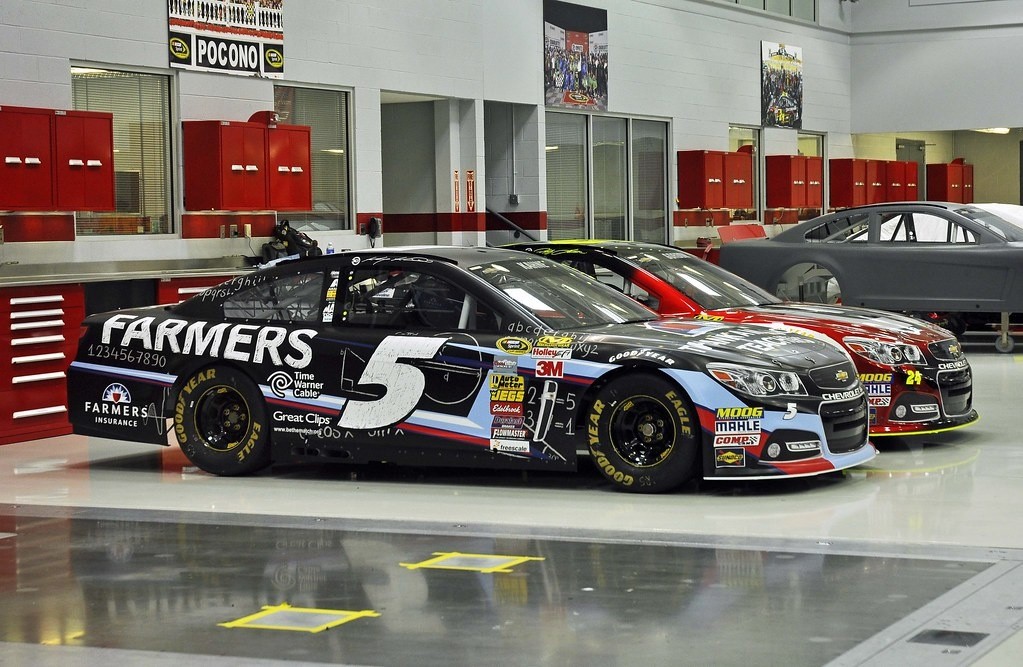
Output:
(326, 242), (335, 254)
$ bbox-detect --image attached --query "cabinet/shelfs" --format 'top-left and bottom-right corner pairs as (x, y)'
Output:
(0, 105), (54, 213)
(49, 109), (117, 212)
(267, 124), (313, 210)
(185, 118), (268, 210)
(676, 149), (975, 210)
(1, 275), (240, 445)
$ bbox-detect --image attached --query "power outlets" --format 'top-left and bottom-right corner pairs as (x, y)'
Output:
(230, 225), (238, 238)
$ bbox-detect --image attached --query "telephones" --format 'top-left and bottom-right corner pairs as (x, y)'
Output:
(370, 218), (381, 239)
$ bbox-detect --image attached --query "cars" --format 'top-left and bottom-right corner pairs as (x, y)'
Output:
(837, 203), (1023, 251)
(702, 199), (1023, 355)
(324, 239), (980, 448)
(63, 245), (879, 493)
